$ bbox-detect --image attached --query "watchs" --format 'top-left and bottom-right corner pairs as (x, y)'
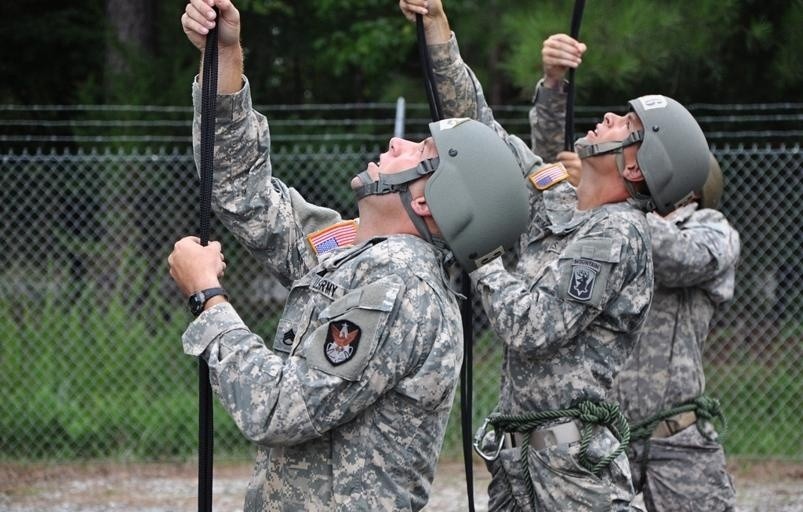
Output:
(188, 287), (229, 317)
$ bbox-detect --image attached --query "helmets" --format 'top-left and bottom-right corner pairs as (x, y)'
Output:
(628, 95), (723, 217)
(424, 118), (529, 273)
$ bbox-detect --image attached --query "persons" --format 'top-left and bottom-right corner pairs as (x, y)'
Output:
(529, 33), (743, 512)
(167, 0), (529, 512)
(399, 0), (710, 512)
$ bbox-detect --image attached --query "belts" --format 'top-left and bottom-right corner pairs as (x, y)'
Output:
(647, 410), (697, 436)
(502, 421), (582, 449)
